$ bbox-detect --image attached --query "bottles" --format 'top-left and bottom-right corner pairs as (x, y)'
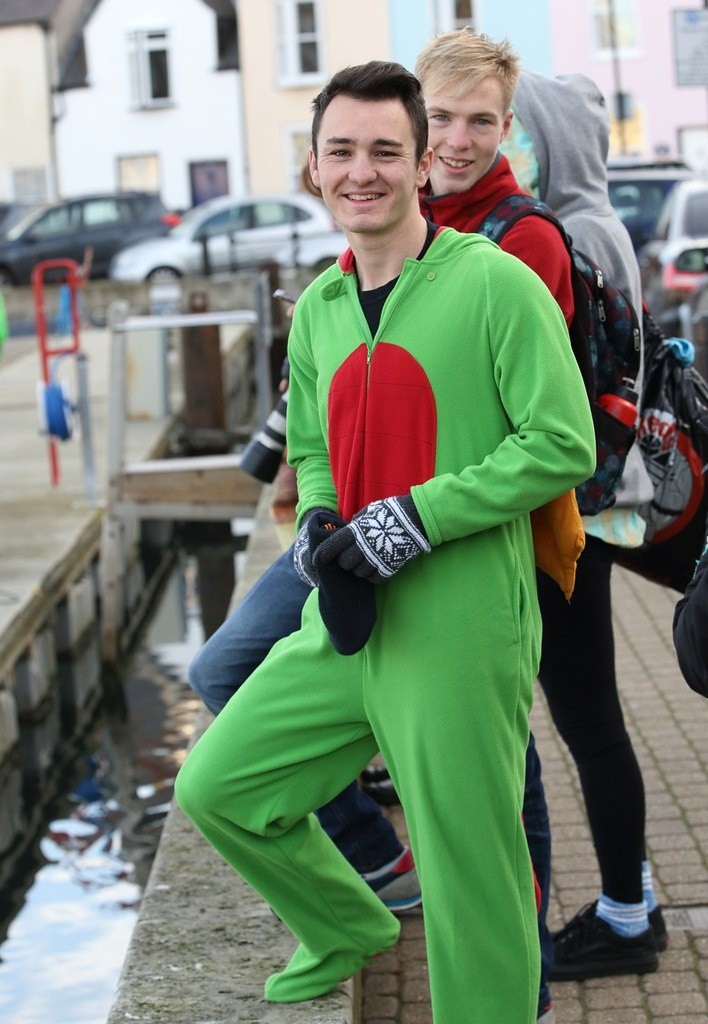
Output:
(580, 377), (639, 517)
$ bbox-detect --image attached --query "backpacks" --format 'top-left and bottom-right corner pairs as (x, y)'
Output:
(477, 194), (642, 517)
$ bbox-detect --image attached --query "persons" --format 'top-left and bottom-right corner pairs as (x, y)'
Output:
(175, 61), (598, 1024)
(187, 30), (574, 1022)
(672, 547), (708, 699)
(500, 70), (669, 985)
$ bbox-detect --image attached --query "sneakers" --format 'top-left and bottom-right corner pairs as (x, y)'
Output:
(644, 903), (670, 953)
(545, 898), (660, 979)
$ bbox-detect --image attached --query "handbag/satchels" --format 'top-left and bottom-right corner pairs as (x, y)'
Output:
(615, 302), (708, 595)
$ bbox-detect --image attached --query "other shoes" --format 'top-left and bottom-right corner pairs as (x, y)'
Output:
(358, 765), (402, 806)
(355, 846), (422, 911)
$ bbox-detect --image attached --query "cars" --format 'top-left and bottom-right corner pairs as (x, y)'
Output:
(516, 156), (708, 377)
(108, 190), (339, 285)
(1, 189), (178, 283)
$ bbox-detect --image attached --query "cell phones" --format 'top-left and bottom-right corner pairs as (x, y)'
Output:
(272, 289), (299, 304)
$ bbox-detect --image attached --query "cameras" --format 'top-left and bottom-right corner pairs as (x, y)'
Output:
(239, 356), (293, 484)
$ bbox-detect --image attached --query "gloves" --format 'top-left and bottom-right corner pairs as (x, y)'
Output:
(294, 507), (348, 588)
(310, 495), (433, 584)
